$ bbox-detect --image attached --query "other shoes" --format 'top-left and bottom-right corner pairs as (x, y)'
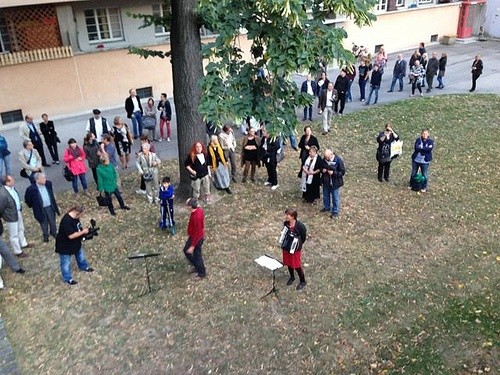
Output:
(85, 268), (94, 272)
(264, 181), (278, 190)
(287, 277), (294, 286)
(378, 176), (392, 183)
(67, 279), (78, 285)
(296, 281), (306, 290)
(320, 208), (338, 220)
(17, 252), (28, 258)
(225, 189), (233, 196)
(17, 269), (26, 274)
(232, 177), (238, 184)
(134, 188), (147, 195)
(241, 178), (257, 184)
(188, 268), (207, 281)
(43, 235), (57, 242)
(110, 206), (131, 218)
(23, 244), (35, 248)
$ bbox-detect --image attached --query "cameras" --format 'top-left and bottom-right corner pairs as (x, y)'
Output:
(83, 219), (100, 241)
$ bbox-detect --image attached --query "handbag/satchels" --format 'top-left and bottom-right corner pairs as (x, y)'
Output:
(390, 139), (403, 158)
(189, 175), (198, 181)
(64, 166), (75, 181)
(97, 191), (110, 207)
(20, 168), (29, 180)
(142, 115), (156, 129)
(276, 150), (284, 163)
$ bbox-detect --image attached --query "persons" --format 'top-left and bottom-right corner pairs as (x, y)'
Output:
(469, 55), (483, 92)
(299, 125), (320, 176)
(376, 123), (400, 182)
(407, 129), (434, 193)
(182, 197), (207, 280)
(320, 148), (345, 218)
(300, 146), (322, 207)
(25, 173), (61, 242)
(234, 63), (298, 188)
(157, 177), (177, 236)
(301, 41), (447, 122)
(19, 112), (61, 183)
(318, 82), (340, 135)
(0, 135), (11, 176)
(185, 122), (236, 203)
(56, 202), (94, 285)
(280, 208), (307, 291)
(62, 88), (172, 215)
(0, 175), (34, 289)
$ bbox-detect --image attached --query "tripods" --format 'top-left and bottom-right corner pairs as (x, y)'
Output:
(255, 256), (284, 299)
(127, 253), (162, 297)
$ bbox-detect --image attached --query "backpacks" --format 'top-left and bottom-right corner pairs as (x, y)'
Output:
(377, 143), (391, 164)
(412, 172), (425, 192)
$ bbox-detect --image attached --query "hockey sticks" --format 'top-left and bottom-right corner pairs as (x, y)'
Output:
(165, 200), (174, 236)
